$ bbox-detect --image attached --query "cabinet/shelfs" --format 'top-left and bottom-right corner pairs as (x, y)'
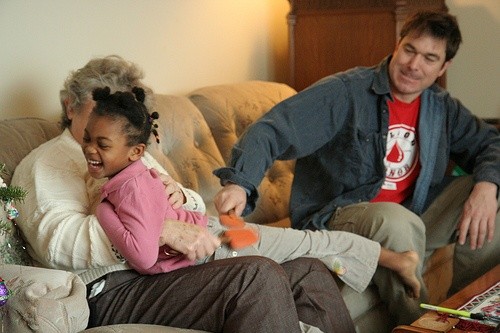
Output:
(289, 0), (450, 92)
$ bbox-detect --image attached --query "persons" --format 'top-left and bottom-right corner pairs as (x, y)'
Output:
(211, 10), (499, 325)
(80, 85), (421, 299)
(8, 53), (357, 333)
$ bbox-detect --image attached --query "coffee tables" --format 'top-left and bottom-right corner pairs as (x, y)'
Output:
(394, 263), (500, 333)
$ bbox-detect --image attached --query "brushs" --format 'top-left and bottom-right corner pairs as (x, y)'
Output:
(219, 209), (260, 253)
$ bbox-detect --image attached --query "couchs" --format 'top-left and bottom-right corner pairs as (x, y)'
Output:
(1, 79), (456, 332)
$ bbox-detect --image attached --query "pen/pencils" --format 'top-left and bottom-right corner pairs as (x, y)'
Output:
(419, 303), (485, 320)
